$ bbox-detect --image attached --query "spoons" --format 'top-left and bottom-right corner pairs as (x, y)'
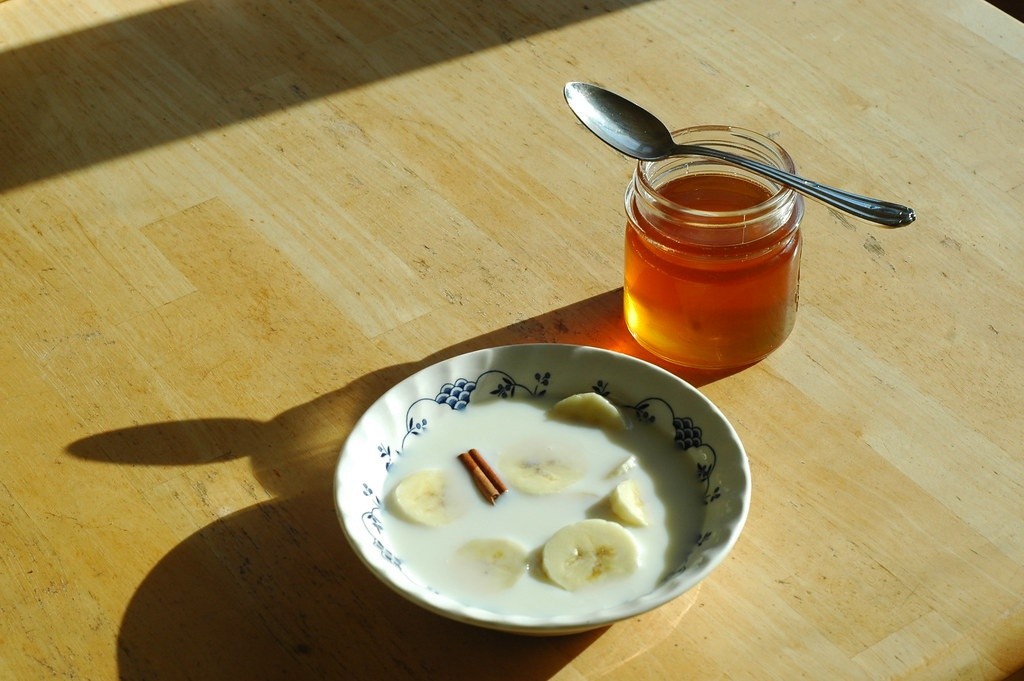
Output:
(565, 81), (917, 232)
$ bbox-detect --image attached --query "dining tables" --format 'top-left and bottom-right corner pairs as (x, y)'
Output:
(2, 0), (1024, 681)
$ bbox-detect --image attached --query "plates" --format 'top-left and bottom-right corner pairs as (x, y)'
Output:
(330, 338), (754, 634)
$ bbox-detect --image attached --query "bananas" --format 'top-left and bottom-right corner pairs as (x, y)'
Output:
(394, 391), (654, 594)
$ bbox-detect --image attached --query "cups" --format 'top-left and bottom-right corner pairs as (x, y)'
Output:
(622, 122), (801, 370)
